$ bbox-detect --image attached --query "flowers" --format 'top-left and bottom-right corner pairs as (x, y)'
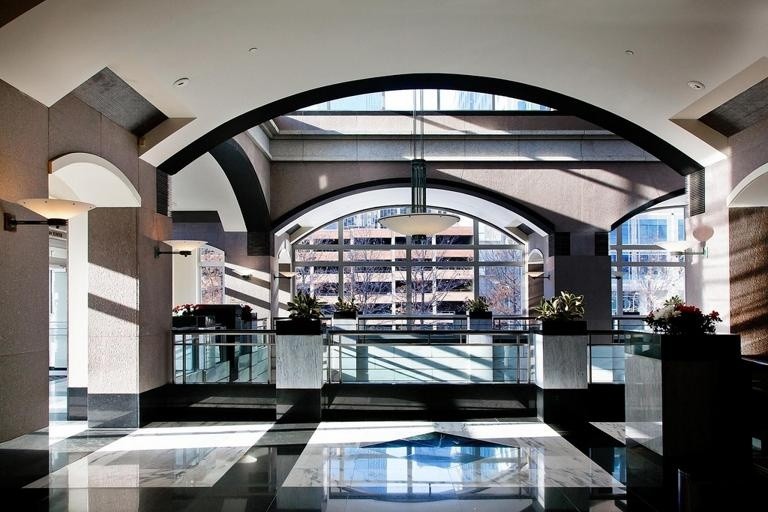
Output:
(529, 291), (586, 318)
(456, 296), (489, 312)
(644, 296), (722, 334)
(172, 304), (201, 316)
(287, 288), (328, 320)
(332, 297), (361, 312)
(240, 304), (254, 314)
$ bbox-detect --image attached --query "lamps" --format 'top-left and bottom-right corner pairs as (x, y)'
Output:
(155, 240), (208, 257)
(4, 198), (96, 232)
(655, 241), (706, 255)
(527, 271), (551, 279)
(377, 89), (461, 240)
